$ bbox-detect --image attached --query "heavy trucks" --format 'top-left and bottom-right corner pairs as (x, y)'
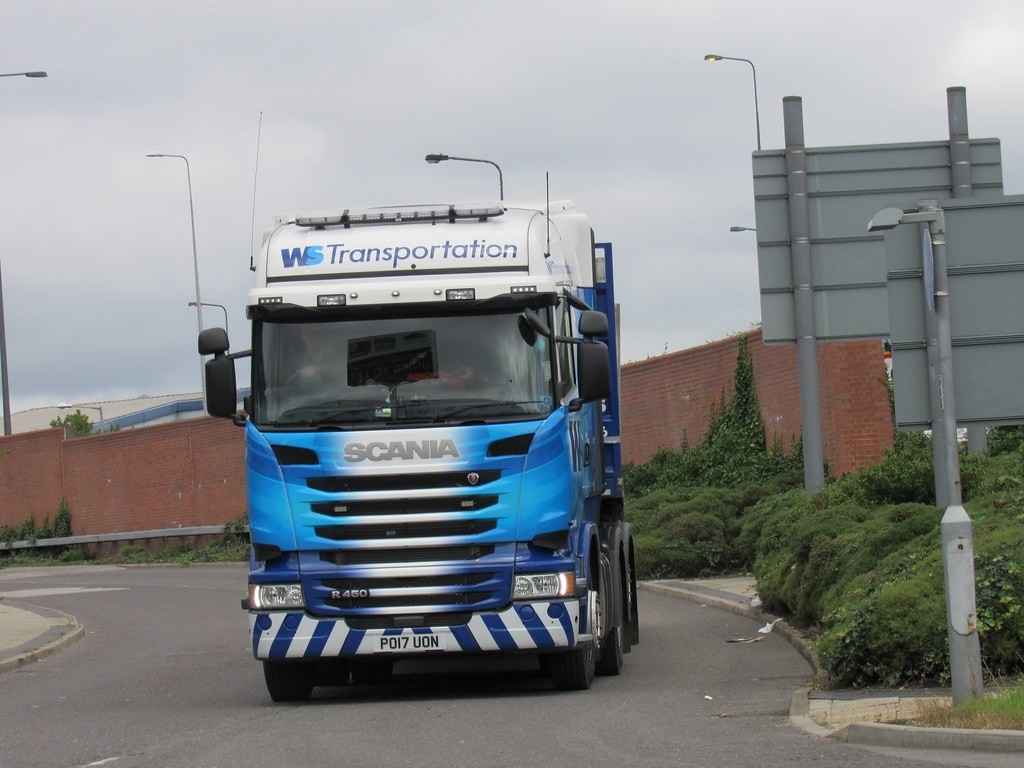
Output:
(199, 197), (637, 707)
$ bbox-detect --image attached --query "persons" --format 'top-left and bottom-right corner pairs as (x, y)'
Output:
(300, 323), (345, 379)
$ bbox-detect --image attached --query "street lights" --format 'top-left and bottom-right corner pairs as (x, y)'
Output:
(0, 71), (49, 436)
(145, 154), (209, 420)
(863, 206), (983, 710)
(57, 404), (105, 434)
(705, 52), (761, 151)
(188, 301), (231, 357)
(425, 154), (506, 203)
